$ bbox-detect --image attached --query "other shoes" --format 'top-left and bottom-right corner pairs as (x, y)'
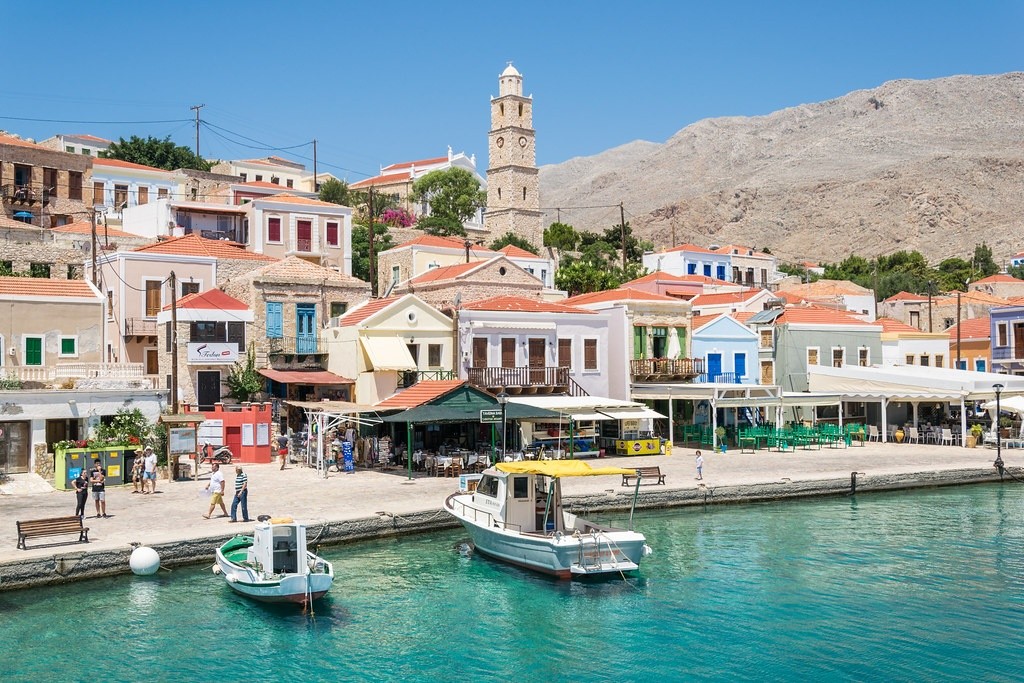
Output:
(97, 514), (101, 518)
(103, 513), (106, 518)
(131, 490), (155, 494)
(220, 514), (228, 517)
(242, 519), (249, 522)
(81, 515), (86, 519)
(228, 520), (237, 522)
(202, 514), (210, 519)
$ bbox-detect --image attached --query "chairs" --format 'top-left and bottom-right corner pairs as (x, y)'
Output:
(682, 422), (1024, 454)
(399, 447), (552, 479)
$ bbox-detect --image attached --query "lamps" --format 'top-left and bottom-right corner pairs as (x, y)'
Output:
(155, 393), (162, 399)
(69, 400), (76, 405)
(21, 184), (28, 192)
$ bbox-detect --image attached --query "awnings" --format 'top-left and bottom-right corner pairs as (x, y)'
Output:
(360, 336), (418, 372)
(556, 410), (614, 421)
(596, 408), (668, 419)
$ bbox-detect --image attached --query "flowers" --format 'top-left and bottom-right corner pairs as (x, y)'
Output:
(52, 408), (149, 449)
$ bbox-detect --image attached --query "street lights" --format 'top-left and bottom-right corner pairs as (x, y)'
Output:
(495, 392), (510, 462)
(991, 384), (1005, 467)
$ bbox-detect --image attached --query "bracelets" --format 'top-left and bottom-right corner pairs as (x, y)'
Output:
(75, 488), (78, 490)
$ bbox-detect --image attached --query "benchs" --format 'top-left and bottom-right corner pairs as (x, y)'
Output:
(621, 466), (666, 487)
(15, 515), (89, 550)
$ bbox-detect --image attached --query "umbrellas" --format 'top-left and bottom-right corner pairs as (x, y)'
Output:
(13, 212), (35, 223)
(981, 395), (1024, 438)
(667, 328), (680, 359)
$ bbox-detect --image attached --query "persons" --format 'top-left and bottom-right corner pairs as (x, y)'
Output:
(132, 448), (145, 493)
(202, 463), (227, 518)
(89, 458), (108, 518)
(275, 431), (291, 470)
(228, 466), (248, 521)
(926, 422), (933, 431)
(695, 450), (703, 480)
(983, 409), (990, 424)
(71, 469), (89, 518)
(142, 446), (157, 494)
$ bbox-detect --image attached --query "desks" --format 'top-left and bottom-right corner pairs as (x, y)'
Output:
(918, 431), (933, 435)
(848, 433), (864, 446)
(465, 455), (491, 473)
(952, 432), (961, 434)
(448, 451), (478, 463)
(547, 450), (565, 460)
(505, 451), (524, 462)
(484, 450), (503, 462)
(425, 457), (465, 477)
(412, 452), (434, 471)
(825, 434), (847, 448)
(741, 438), (755, 453)
(797, 435), (820, 451)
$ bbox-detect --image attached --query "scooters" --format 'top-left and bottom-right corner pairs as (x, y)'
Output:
(196, 441), (234, 464)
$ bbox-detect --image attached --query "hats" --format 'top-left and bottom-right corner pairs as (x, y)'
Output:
(134, 448), (144, 454)
(144, 446), (154, 453)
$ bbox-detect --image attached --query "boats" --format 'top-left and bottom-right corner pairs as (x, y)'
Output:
(442, 456), (654, 580)
(213, 513), (335, 605)
